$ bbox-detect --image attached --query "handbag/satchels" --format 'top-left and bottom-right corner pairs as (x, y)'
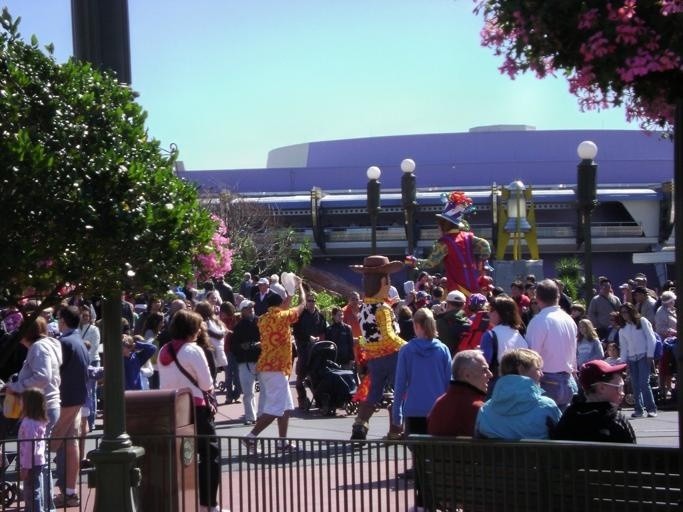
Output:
(202, 392), (218, 415)
(2, 372), (23, 420)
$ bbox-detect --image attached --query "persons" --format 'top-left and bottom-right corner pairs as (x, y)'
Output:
(403, 192), (491, 296)
(0, 255), (680, 510)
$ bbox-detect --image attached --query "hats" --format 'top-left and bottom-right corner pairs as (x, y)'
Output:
(349, 256), (404, 273)
(281, 271), (298, 296)
(240, 300), (256, 309)
(636, 272), (677, 304)
(447, 290), (467, 303)
(417, 291), (430, 298)
(436, 199), (466, 228)
(578, 360), (628, 385)
(170, 310), (203, 338)
(257, 277), (269, 285)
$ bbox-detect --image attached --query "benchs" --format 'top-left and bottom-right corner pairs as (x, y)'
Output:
(401, 431), (681, 512)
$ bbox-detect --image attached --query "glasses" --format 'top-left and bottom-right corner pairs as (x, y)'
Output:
(306, 299), (316, 302)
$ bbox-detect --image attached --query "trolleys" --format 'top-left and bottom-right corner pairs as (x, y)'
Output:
(0, 370), (26, 511)
(216, 380), (260, 393)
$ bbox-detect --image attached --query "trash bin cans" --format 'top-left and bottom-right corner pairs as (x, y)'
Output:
(124, 386), (200, 512)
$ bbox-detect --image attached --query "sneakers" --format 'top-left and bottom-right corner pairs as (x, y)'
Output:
(200, 505), (231, 512)
(395, 467), (415, 481)
(241, 434), (265, 455)
(51, 492), (82, 508)
(276, 440), (305, 453)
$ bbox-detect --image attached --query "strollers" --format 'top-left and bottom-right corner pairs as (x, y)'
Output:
(300, 338), (383, 416)
(621, 366), (662, 406)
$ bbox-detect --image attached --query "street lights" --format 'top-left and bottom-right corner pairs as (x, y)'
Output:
(398, 156), (420, 258)
(363, 163), (383, 255)
(572, 137), (600, 309)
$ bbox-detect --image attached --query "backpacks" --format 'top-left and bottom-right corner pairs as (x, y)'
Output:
(654, 333), (664, 360)
